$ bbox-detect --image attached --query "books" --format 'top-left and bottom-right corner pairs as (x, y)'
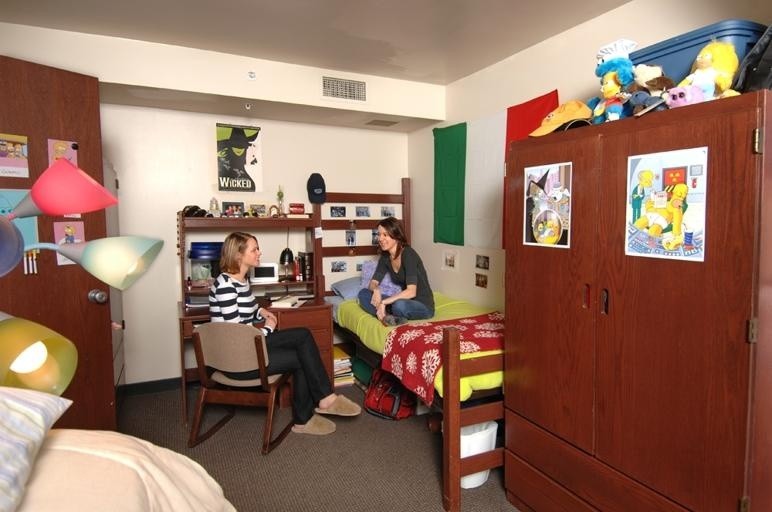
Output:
(270, 296), (299, 308)
(287, 214), (309, 218)
(333, 344), (356, 387)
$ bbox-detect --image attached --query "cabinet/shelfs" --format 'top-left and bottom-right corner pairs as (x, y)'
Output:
(177, 212), (324, 308)
(505, 88), (772, 512)
(102, 155), (127, 420)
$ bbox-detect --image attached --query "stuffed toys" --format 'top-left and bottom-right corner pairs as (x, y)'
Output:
(592, 38), (742, 123)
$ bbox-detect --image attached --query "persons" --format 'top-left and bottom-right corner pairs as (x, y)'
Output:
(357, 216), (434, 326)
(225, 204), (242, 215)
(210, 233), (361, 436)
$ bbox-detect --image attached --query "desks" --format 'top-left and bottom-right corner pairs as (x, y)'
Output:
(177, 301), (333, 424)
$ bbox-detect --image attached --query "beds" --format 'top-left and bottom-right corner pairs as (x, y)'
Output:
(14, 431), (237, 512)
(311, 176), (503, 510)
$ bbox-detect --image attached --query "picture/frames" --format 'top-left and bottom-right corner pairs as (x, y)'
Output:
(250, 204), (267, 216)
(222, 202), (244, 217)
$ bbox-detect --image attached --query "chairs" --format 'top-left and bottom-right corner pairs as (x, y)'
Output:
(190, 324), (293, 454)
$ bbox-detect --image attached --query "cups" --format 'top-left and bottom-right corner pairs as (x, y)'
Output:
(684, 231), (695, 248)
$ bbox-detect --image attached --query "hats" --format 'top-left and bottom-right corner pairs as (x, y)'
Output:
(528, 100), (592, 137)
(307, 173), (326, 203)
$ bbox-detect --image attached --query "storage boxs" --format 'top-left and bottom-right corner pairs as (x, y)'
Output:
(629, 19), (765, 89)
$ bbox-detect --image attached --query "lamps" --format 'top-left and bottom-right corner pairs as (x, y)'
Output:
(0, 160), (165, 396)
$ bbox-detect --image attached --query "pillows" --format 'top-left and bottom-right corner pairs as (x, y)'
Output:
(331, 276), (362, 298)
(0, 387), (73, 512)
(362, 261), (401, 295)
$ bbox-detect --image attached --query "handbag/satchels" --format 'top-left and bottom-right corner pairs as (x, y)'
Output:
(364, 366), (417, 420)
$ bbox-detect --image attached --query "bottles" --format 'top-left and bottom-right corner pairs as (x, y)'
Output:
(187, 277), (192, 291)
(209, 196), (219, 210)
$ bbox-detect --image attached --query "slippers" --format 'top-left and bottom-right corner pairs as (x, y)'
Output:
(314, 394), (362, 416)
(291, 414), (336, 435)
